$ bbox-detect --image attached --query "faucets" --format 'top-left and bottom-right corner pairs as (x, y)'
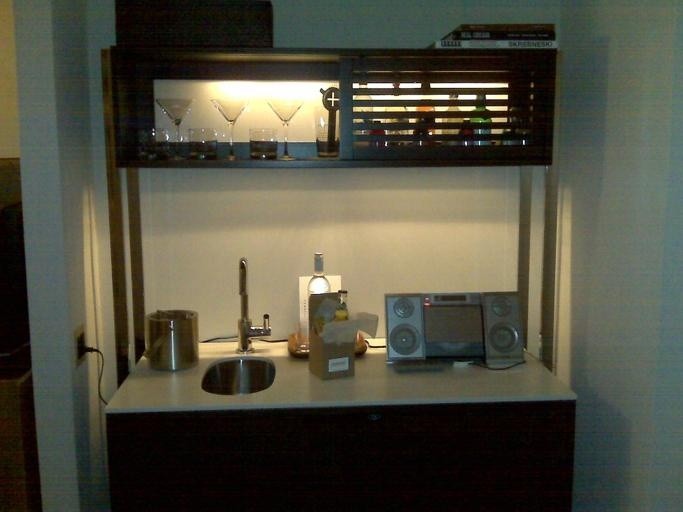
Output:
(236, 258), (272, 352)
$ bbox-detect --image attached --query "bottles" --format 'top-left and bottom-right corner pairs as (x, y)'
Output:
(386, 82), (409, 149)
(471, 93), (493, 146)
(503, 106), (523, 145)
(443, 93), (464, 150)
(352, 70), (374, 149)
(306, 253), (331, 303)
(458, 129), (474, 147)
(331, 290), (349, 322)
(369, 121), (386, 152)
(414, 83), (436, 147)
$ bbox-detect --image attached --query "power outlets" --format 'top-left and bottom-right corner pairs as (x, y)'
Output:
(74, 327), (87, 368)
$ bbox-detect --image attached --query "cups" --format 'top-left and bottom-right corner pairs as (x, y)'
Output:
(314, 115), (340, 160)
(139, 128), (170, 160)
(189, 127), (217, 161)
(249, 128), (278, 161)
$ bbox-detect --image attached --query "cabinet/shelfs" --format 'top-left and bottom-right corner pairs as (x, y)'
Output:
(104, 48), (557, 167)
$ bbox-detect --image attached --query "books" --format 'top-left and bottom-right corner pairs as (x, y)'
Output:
(426, 23), (559, 51)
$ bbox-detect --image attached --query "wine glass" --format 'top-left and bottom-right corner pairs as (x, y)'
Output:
(156, 98), (195, 161)
(267, 99), (305, 160)
(210, 99), (253, 162)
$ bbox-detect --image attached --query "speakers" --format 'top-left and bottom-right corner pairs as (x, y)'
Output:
(384, 293), (426, 364)
(482, 291), (525, 369)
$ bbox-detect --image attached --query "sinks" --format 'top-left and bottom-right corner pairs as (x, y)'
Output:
(202, 355), (276, 395)
